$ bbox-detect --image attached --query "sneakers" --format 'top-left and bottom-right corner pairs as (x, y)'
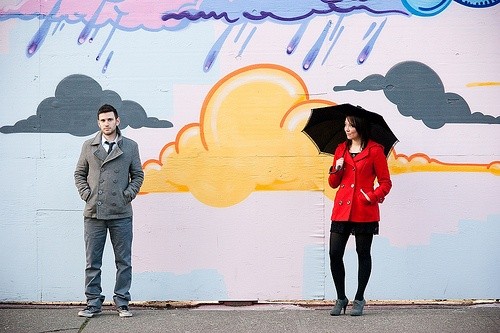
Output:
(118, 306), (133, 317)
(78, 306), (102, 318)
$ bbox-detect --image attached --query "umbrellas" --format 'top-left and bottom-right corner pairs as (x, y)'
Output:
(301, 103), (400, 174)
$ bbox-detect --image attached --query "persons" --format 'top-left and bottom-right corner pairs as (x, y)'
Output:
(74, 104), (144, 318)
(328, 114), (393, 316)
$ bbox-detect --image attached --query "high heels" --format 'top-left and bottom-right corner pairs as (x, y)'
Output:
(350, 298), (366, 316)
(330, 297), (348, 316)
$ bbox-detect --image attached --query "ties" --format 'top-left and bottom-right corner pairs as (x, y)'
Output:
(105, 141), (116, 155)
